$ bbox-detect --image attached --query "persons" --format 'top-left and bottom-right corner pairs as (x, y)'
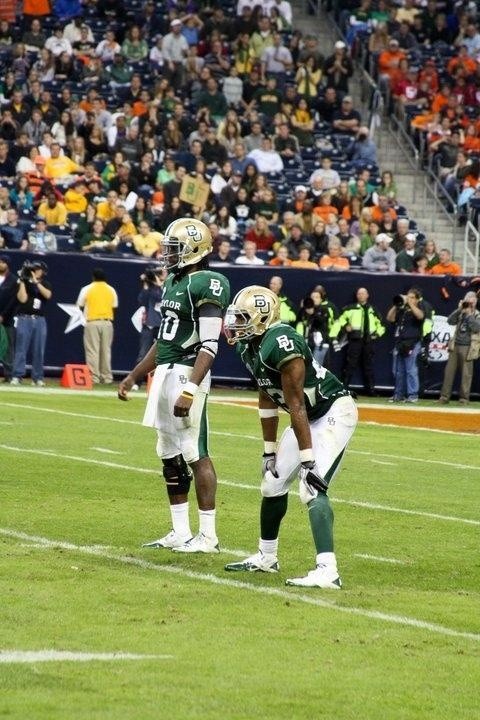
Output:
(221, 285), (360, 591)
(1, 1), (480, 275)
(0, 261), (480, 407)
(118, 218), (230, 555)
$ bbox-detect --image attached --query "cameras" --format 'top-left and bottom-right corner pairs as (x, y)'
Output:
(461, 300), (471, 310)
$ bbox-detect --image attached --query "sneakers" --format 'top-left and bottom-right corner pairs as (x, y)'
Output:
(285, 564), (341, 589)
(142, 531), (193, 547)
(171, 532), (220, 554)
(31, 379), (45, 387)
(131, 384), (139, 391)
(10, 377), (20, 385)
(387, 396), (405, 402)
(433, 398), (470, 406)
(224, 551), (281, 572)
(406, 396), (418, 402)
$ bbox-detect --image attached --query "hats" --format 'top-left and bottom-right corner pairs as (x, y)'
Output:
(170, 18), (184, 27)
(34, 154), (46, 166)
(294, 185), (306, 193)
(375, 233), (393, 243)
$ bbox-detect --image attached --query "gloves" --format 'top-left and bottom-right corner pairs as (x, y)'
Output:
(298, 461), (328, 496)
(262, 454), (279, 479)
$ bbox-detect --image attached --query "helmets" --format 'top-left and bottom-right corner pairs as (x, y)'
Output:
(160, 218), (214, 270)
(224, 284), (282, 344)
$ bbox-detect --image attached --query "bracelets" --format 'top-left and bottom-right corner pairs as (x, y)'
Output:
(263, 440), (278, 455)
(182, 394), (193, 399)
(299, 449), (314, 462)
(182, 382), (199, 396)
